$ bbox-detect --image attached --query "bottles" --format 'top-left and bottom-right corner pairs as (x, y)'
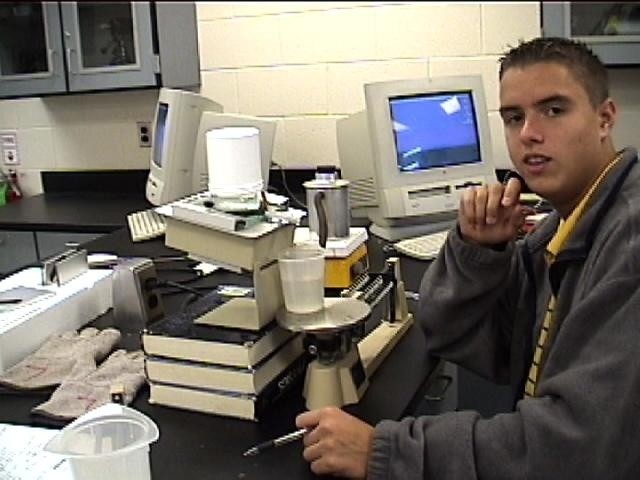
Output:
(98, 19), (134, 66)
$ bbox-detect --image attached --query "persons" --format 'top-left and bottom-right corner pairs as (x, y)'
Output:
(293, 36), (639, 480)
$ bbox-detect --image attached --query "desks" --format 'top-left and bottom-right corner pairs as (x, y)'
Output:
(0, 191), (435, 480)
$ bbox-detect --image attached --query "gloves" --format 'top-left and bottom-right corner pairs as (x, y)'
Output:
(0, 327), (121, 389)
(32, 350), (148, 418)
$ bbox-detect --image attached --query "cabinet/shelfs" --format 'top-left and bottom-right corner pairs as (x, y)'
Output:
(539, 1), (640, 69)
(0, 1), (201, 99)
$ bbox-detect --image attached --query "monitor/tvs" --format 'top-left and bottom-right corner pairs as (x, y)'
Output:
(335, 73), (495, 241)
(145, 87), (278, 206)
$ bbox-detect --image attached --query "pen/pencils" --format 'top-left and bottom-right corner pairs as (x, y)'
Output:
(243, 429), (308, 456)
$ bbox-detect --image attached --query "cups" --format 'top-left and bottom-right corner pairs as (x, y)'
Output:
(276, 247), (328, 313)
(304, 178), (349, 245)
(40, 404), (159, 479)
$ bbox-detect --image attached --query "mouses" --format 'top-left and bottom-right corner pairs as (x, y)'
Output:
(88, 252), (118, 268)
(525, 212), (550, 226)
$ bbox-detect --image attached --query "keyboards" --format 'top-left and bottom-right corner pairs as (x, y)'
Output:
(392, 229), (452, 261)
(126, 207), (167, 243)
